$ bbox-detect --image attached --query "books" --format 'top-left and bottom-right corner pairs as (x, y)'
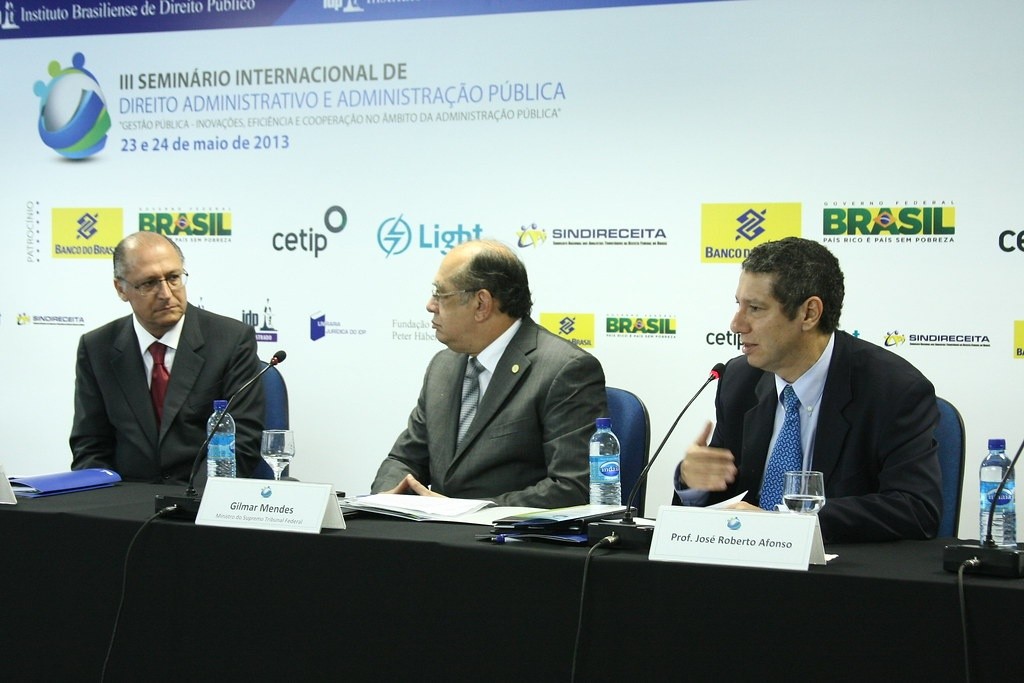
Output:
(338, 493), (549, 526)
(8, 468), (122, 500)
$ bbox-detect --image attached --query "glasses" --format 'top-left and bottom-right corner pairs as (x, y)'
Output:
(433, 288), (495, 305)
(118, 268), (189, 296)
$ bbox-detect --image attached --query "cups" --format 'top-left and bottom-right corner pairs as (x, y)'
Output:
(784, 471), (826, 516)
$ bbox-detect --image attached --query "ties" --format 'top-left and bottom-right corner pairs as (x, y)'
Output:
(759, 386), (802, 513)
(455, 356), (486, 453)
(148, 341), (170, 420)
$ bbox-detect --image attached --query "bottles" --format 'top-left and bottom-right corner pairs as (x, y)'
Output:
(589, 418), (621, 523)
(207, 401), (236, 478)
(978, 439), (1018, 554)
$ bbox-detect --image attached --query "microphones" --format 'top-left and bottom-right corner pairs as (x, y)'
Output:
(155, 350), (287, 522)
(586, 362), (726, 550)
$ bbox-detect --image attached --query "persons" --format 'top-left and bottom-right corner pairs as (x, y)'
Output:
(671, 237), (943, 545)
(370, 240), (609, 509)
(69, 231), (265, 486)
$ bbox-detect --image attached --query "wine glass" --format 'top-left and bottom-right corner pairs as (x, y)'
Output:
(261, 430), (296, 480)
